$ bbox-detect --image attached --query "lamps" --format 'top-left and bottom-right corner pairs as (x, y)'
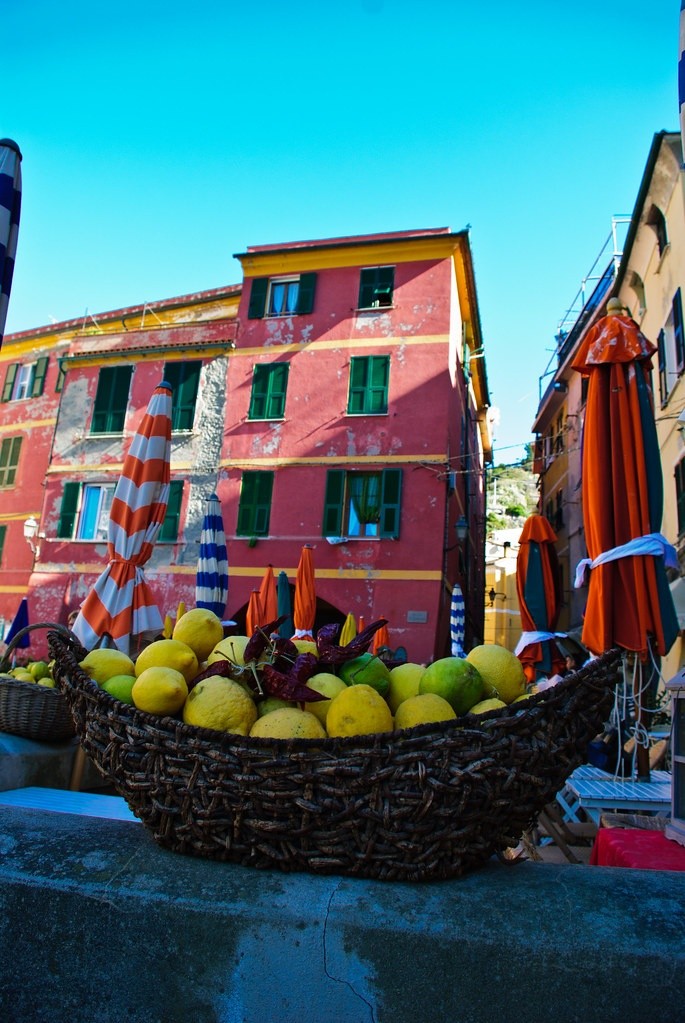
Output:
(23, 514), (38, 553)
(447, 515), (469, 552)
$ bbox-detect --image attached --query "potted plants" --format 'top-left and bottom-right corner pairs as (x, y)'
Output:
(364, 505), (380, 536)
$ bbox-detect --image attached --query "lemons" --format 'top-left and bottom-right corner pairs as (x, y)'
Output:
(74, 608), (546, 754)
(0, 659), (55, 689)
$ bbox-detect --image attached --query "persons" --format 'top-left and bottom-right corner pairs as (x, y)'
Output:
(562, 652), (581, 678)
(67, 610), (79, 629)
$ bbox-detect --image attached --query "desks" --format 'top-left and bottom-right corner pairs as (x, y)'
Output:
(554, 766), (672, 806)
(588, 829), (685, 871)
(540, 780), (672, 847)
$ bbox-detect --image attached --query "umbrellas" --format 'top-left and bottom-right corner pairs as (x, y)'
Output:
(338, 611), (357, 647)
(514, 515), (563, 685)
(72, 384), (173, 800)
(570, 295), (679, 785)
(195, 492), (228, 620)
(451, 584), (465, 657)
(260, 563), (278, 635)
(293, 544), (316, 642)
(277, 569), (294, 638)
(4, 597), (31, 669)
(358, 616), (364, 633)
(247, 588), (264, 638)
(373, 614), (390, 654)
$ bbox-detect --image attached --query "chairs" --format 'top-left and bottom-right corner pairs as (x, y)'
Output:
(512, 806), (600, 864)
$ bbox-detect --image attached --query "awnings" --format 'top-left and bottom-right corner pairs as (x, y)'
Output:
(669, 576), (685, 630)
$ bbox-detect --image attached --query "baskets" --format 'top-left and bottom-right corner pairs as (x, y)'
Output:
(0, 622), (84, 738)
(47, 631), (623, 880)
